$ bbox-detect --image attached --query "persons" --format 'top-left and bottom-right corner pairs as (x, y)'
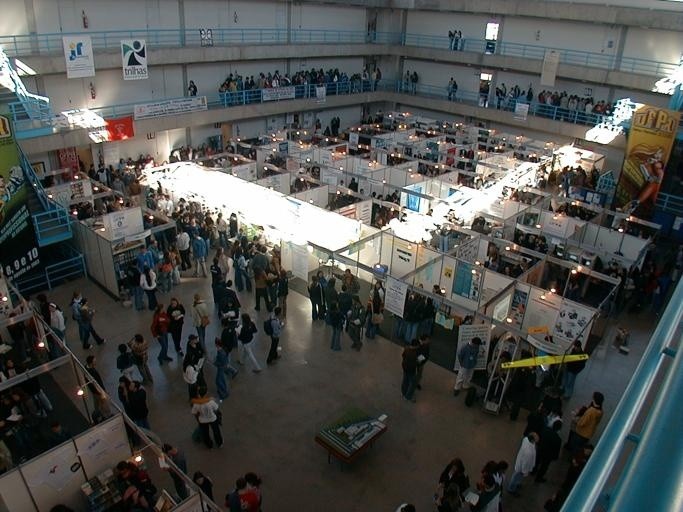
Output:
(448, 29), (465, 51)
(0, 67), (683, 512)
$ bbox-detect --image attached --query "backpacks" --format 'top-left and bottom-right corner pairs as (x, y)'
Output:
(326, 313), (338, 325)
(233, 260), (239, 269)
(264, 319), (273, 335)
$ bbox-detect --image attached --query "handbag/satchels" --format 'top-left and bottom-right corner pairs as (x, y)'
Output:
(372, 313), (383, 324)
(201, 316), (209, 326)
